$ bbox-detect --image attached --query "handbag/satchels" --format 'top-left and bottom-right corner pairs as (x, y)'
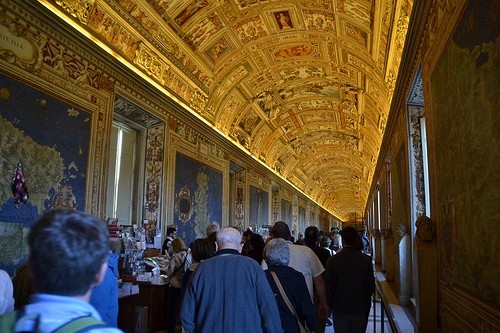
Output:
(296, 318), (310, 333)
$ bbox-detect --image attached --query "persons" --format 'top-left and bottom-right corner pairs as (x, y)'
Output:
(0, 209), (121, 333)
(160, 221), (375, 333)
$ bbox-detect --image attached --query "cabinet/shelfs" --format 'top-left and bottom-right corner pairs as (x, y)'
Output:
(116, 279), (182, 333)
